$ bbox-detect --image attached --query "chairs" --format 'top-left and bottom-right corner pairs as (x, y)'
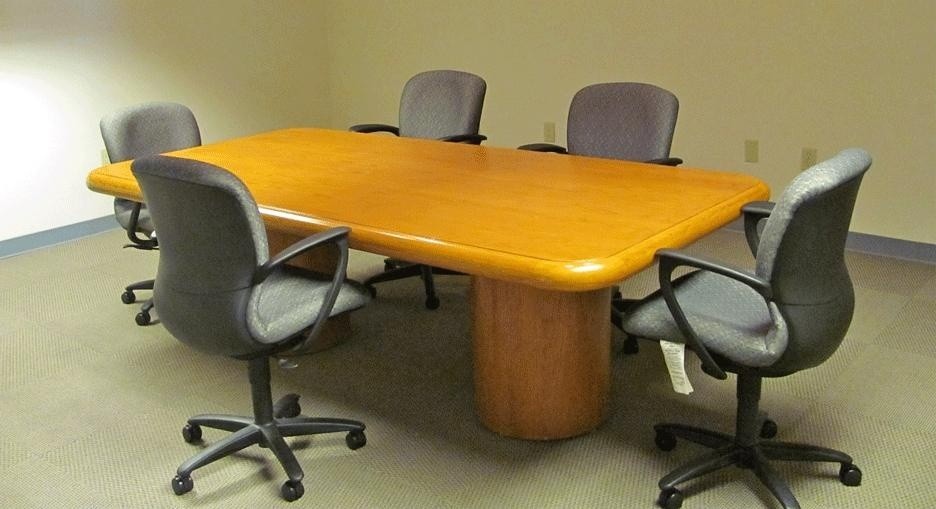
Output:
(132, 158), (369, 499)
(515, 80), (686, 357)
(101, 101), (203, 323)
(351, 70), (488, 307)
(624, 147), (874, 509)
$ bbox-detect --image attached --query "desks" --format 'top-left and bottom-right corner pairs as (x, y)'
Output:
(89, 128), (771, 440)
